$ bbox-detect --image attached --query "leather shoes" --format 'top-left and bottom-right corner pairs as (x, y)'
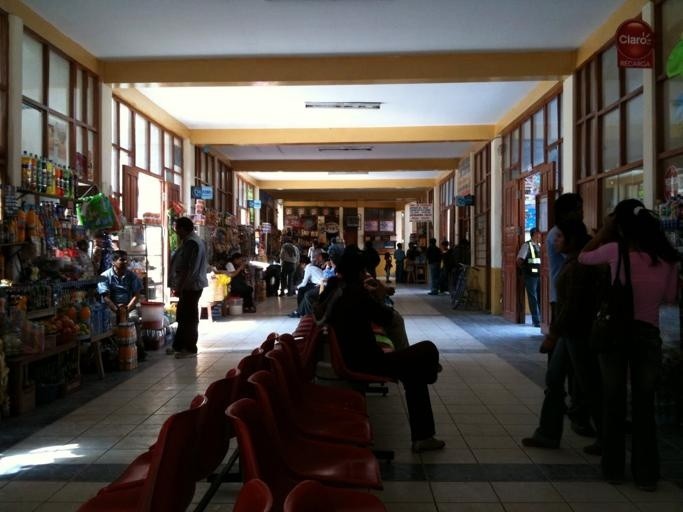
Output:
(570, 420), (597, 439)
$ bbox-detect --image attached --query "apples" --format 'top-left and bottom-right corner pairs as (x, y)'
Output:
(45, 316), (90, 336)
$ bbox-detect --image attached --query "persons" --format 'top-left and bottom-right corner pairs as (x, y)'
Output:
(223, 252), (257, 313)
(543, 192), (599, 438)
(96, 250), (148, 365)
(521, 212), (611, 454)
(164, 217), (208, 359)
(574, 198), (680, 491)
(7, 237), (37, 285)
(264, 238), (450, 455)
(516, 227), (543, 327)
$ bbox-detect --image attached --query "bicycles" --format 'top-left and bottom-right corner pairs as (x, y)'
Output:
(445, 261), (479, 310)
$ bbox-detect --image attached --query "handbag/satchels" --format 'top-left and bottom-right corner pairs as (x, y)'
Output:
(593, 276), (636, 359)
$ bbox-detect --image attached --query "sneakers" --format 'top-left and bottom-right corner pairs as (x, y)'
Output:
(174, 351), (197, 359)
(521, 433), (559, 450)
(411, 438), (445, 454)
(582, 439), (603, 457)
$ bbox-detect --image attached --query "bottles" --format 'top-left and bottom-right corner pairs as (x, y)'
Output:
(655, 193), (683, 248)
(0, 319), (46, 354)
(141, 327), (175, 352)
(19, 201), (86, 250)
(92, 301), (139, 370)
(21, 150), (75, 198)
(0, 282), (78, 317)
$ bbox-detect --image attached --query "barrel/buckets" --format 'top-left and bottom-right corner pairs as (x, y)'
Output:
(229, 299), (243, 316)
(141, 301), (165, 329)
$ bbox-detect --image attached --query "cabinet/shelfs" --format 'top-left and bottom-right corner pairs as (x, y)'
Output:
(0, 181), (111, 414)
